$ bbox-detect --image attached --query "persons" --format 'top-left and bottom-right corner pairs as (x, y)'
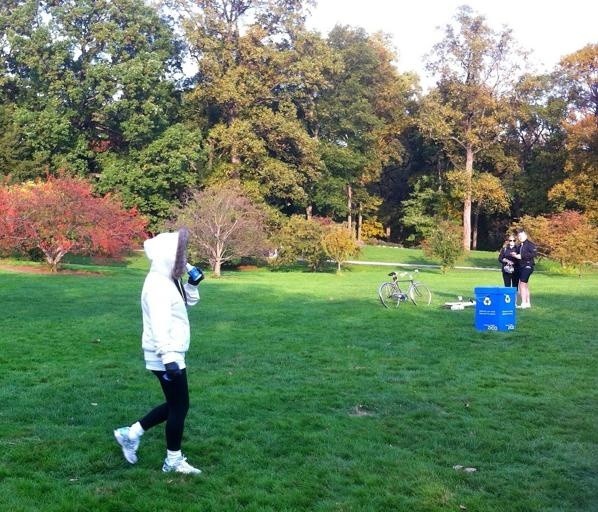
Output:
(498, 228), (537, 308)
(114, 229), (202, 474)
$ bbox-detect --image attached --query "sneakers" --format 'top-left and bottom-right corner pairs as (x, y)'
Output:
(114, 426), (141, 465)
(162, 454), (201, 475)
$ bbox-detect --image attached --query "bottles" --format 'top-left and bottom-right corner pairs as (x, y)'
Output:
(185, 263), (201, 282)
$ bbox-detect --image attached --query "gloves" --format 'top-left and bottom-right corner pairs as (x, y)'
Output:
(188, 267), (204, 286)
(164, 362), (182, 379)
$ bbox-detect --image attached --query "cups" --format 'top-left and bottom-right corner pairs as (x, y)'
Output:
(458, 295), (462, 302)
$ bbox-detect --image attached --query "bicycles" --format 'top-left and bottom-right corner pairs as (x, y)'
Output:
(377, 268), (432, 309)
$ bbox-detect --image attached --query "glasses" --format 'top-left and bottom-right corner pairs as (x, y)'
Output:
(509, 240), (516, 242)
